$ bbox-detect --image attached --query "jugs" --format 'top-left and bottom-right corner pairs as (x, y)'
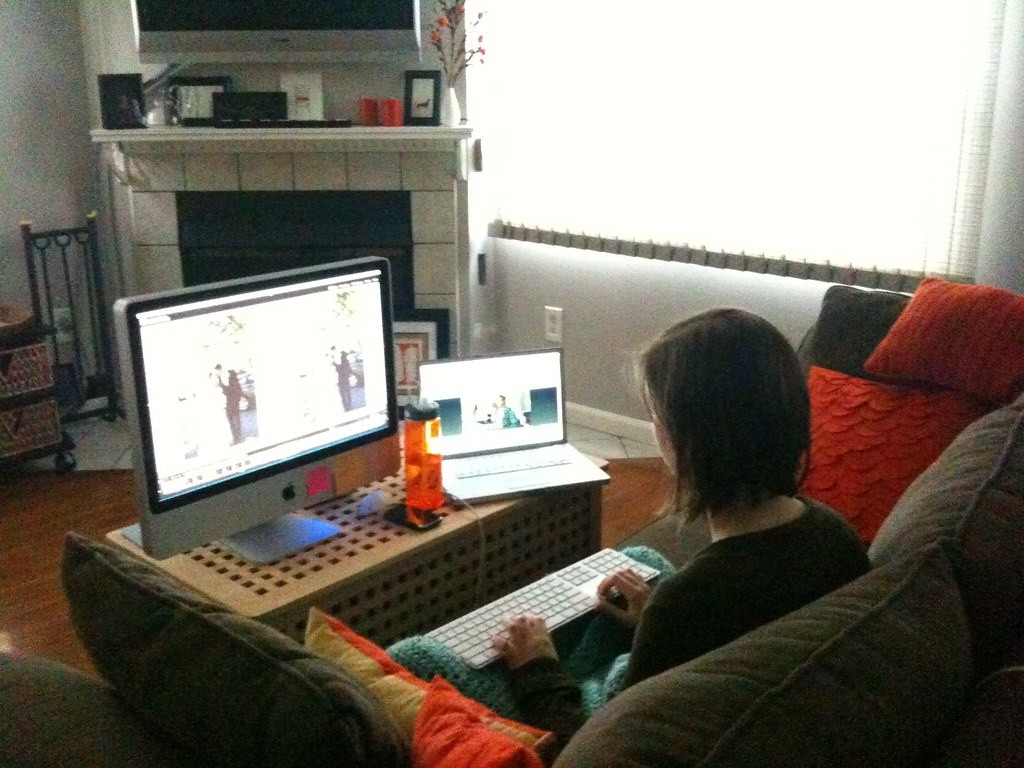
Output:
(131, 85), (180, 126)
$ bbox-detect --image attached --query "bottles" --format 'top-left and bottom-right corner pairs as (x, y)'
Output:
(403, 400), (442, 528)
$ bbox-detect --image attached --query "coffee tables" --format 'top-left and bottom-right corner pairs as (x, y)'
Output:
(104, 435), (609, 647)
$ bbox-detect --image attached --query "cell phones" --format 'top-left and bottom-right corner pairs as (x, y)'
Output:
(384, 505), (443, 531)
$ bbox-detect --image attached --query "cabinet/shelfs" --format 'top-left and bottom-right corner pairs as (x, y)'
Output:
(0, 326), (76, 472)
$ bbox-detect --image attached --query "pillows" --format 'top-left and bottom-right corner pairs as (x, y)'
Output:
(60, 530), (405, 768)
(300, 609), (552, 756)
(407, 674), (541, 766)
(795, 366), (998, 542)
(865, 279), (1023, 398)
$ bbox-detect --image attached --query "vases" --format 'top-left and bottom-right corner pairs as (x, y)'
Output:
(439, 88), (461, 126)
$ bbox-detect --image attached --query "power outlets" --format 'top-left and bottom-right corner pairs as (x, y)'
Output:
(545, 305), (564, 346)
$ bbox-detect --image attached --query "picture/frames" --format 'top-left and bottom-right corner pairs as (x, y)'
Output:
(166, 75), (230, 126)
(402, 70), (440, 126)
(391, 307), (450, 413)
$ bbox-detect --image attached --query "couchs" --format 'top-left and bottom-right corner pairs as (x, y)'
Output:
(0, 285), (1024, 767)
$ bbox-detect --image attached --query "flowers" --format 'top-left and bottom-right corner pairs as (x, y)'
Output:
(428, 0), (485, 81)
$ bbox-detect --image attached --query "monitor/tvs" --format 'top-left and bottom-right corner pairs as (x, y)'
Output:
(130, 0), (421, 68)
(114, 255), (398, 566)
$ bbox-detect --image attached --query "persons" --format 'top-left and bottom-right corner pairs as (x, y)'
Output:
(211, 362), (249, 446)
(329, 347), (361, 413)
(384, 308), (866, 766)
(491, 394), (520, 429)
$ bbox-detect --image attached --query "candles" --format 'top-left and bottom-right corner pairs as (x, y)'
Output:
(381, 97), (401, 127)
(359, 96), (377, 126)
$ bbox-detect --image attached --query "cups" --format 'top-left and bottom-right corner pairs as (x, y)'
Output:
(173, 74), (232, 126)
(360, 96), (378, 126)
(377, 98), (403, 127)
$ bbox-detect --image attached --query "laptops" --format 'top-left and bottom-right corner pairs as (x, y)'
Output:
(417, 347), (610, 507)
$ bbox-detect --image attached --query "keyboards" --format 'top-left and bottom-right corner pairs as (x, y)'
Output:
(425, 548), (661, 668)
